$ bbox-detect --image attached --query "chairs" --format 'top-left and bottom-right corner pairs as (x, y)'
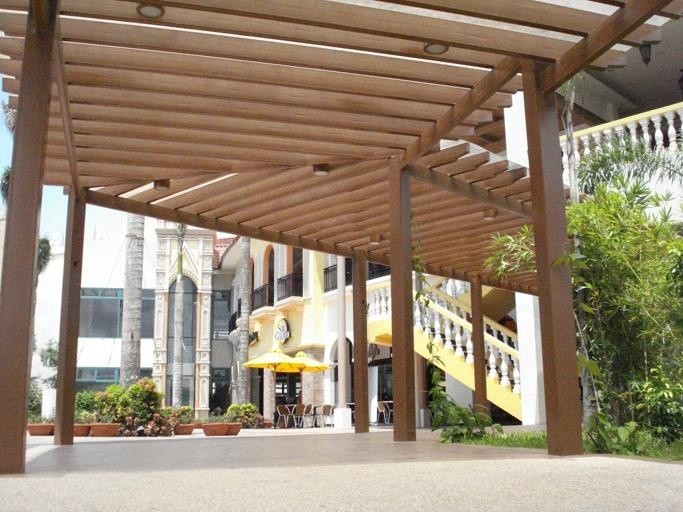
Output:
(273, 398), (395, 430)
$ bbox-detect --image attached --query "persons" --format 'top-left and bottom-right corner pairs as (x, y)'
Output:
(296, 391), (301, 405)
(381, 393), (392, 410)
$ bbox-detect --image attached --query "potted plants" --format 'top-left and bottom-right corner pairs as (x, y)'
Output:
(23, 374), (242, 440)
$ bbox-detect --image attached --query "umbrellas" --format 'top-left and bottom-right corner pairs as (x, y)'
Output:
(243, 344), (305, 413)
(292, 351), (330, 404)
